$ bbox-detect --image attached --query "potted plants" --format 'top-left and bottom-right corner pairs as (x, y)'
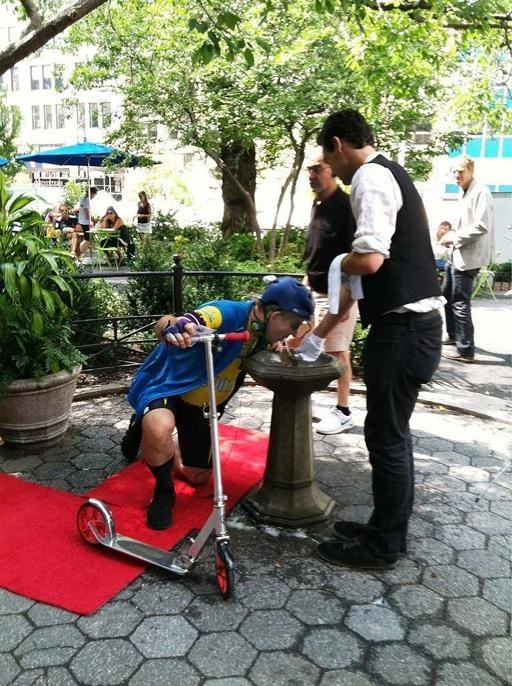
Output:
(0, 172), (89, 451)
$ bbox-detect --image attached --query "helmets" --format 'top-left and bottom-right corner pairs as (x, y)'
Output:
(259, 275), (316, 319)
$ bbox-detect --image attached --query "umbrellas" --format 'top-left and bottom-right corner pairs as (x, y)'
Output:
(15, 136), (162, 255)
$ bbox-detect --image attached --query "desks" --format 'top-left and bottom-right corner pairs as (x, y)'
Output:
(85, 231), (122, 271)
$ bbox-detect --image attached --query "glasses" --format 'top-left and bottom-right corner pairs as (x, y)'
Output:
(301, 167), (332, 173)
(288, 320), (300, 331)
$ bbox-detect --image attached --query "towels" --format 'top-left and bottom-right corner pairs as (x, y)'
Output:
(326, 253), (364, 315)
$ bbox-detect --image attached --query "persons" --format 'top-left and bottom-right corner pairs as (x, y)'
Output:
(44, 184), (97, 261)
(127, 275), (316, 530)
(291, 109), (448, 572)
(430, 220), (453, 272)
(98, 206), (130, 266)
(295, 152), (359, 437)
(435, 154), (493, 364)
(133, 189), (153, 252)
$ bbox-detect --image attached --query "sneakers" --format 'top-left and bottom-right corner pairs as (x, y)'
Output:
(315, 518), (408, 569)
(442, 347), (477, 364)
(121, 412), (142, 461)
(439, 336), (456, 345)
(313, 405), (355, 436)
(145, 483), (179, 530)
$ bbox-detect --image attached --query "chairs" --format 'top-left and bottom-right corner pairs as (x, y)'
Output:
(91, 235), (120, 271)
(470, 260), (497, 301)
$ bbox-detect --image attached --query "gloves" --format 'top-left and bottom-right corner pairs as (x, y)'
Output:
(294, 334), (326, 363)
(160, 317), (195, 341)
